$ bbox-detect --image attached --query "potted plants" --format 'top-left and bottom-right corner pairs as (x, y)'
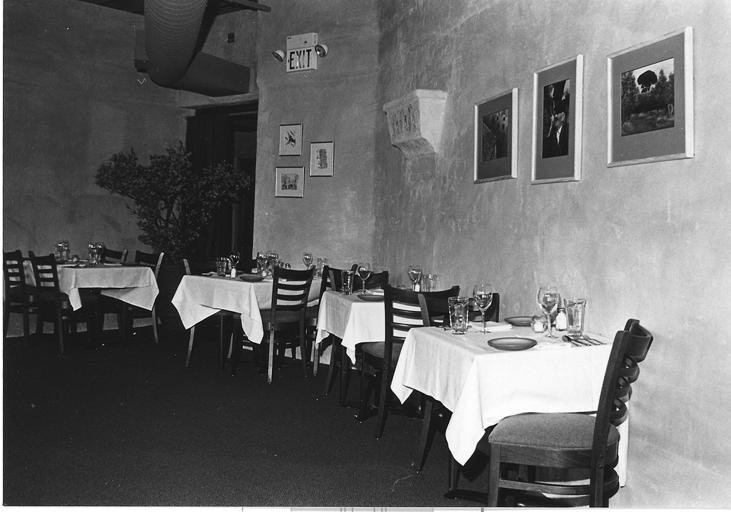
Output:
(93, 139), (255, 317)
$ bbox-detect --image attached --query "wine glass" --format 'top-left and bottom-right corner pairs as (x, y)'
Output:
(302, 252), (313, 269)
(473, 283), (493, 335)
(537, 287), (560, 340)
(357, 263), (371, 294)
(407, 265), (421, 291)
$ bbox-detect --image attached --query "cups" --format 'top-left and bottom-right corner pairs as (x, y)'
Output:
(56, 240), (70, 263)
(317, 257), (328, 275)
(216, 257), (227, 276)
(228, 250), (241, 266)
(256, 252), (279, 274)
(341, 270), (355, 295)
(422, 273), (436, 292)
(448, 297), (470, 335)
(564, 298), (586, 336)
(89, 241), (104, 264)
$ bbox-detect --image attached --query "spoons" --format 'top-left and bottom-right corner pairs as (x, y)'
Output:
(583, 334), (607, 345)
(562, 334), (581, 348)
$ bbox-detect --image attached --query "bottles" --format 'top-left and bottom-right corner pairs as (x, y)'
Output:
(533, 318), (546, 332)
(230, 266), (236, 278)
(285, 263), (291, 269)
(556, 309), (566, 330)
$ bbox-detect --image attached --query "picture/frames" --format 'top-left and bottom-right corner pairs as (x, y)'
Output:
(606, 26), (695, 168)
(531, 55), (584, 184)
(470, 88), (518, 184)
(275, 166), (305, 198)
(310, 142), (335, 177)
(279, 124), (303, 156)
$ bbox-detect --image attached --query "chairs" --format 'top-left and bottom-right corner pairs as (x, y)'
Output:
(324, 269), (390, 406)
(173, 250), (358, 384)
(486, 316), (653, 507)
(353, 284), (461, 439)
(5, 241), (168, 356)
(413, 291), (503, 494)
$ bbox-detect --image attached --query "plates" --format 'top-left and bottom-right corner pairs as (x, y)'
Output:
(358, 294), (384, 300)
(239, 274), (264, 282)
(488, 337), (538, 352)
(504, 315), (532, 326)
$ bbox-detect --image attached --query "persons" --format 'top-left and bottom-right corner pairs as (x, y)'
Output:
(543, 99), (568, 158)
